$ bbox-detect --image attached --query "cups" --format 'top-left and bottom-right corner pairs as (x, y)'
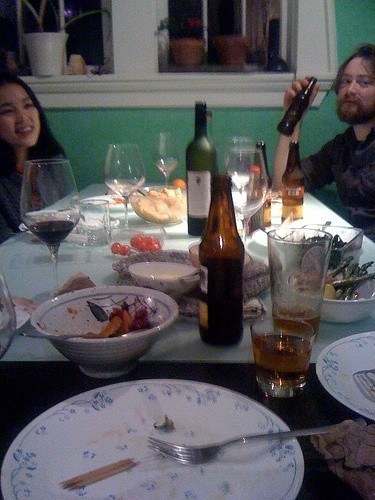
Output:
(77, 200), (110, 248)
(0, 270), (17, 359)
(250, 318), (315, 398)
(267, 228), (332, 340)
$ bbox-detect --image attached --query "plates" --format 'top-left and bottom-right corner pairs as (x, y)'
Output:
(1, 379), (304, 500)
(19, 212), (120, 234)
(316, 331), (375, 418)
(80, 195), (134, 209)
(12, 299), (29, 330)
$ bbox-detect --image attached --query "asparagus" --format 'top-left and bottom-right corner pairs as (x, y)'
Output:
(328, 256), (375, 299)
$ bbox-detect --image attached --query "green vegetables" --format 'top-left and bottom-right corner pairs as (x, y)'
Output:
(298, 234), (347, 275)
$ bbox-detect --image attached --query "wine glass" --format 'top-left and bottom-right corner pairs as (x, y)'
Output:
(155, 132), (179, 189)
(224, 136), (268, 244)
(20, 159), (81, 297)
(105, 143), (146, 230)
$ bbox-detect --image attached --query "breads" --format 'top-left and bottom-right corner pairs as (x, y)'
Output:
(136, 187), (187, 221)
(59, 272), (96, 294)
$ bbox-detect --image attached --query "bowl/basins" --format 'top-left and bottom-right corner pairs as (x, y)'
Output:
(30, 285), (179, 378)
(190, 245), (252, 271)
(282, 224), (363, 267)
(130, 185), (186, 225)
(129, 262), (199, 298)
(289, 269), (375, 324)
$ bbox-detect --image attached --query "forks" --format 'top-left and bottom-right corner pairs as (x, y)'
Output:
(147, 424), (330, 465)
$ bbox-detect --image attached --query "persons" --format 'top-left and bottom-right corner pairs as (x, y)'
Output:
(0, 65), (78, 243)
(273, 45), (375, 244)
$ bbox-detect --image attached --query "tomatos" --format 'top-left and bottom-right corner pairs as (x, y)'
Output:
(111, 234), (162, 255)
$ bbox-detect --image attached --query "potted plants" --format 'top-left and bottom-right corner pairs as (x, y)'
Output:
(158, 8), (250, 69)
(22, 3), (112, 76)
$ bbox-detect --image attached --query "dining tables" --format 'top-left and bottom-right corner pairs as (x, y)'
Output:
(0, 183), (375, 500)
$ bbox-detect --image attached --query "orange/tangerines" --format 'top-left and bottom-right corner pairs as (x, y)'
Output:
(171, 179), (186, 189)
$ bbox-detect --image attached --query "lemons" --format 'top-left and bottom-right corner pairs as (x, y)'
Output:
(324, 284), (336, 299)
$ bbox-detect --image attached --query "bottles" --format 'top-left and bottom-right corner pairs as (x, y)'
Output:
(246, 167), (264, 235)
(281, 141), (305, 223)
(254, 141), (273, 227)
(199, 175), (245, 348)
(276, 76), (317, 136)
(185, 100), (218, 236)
